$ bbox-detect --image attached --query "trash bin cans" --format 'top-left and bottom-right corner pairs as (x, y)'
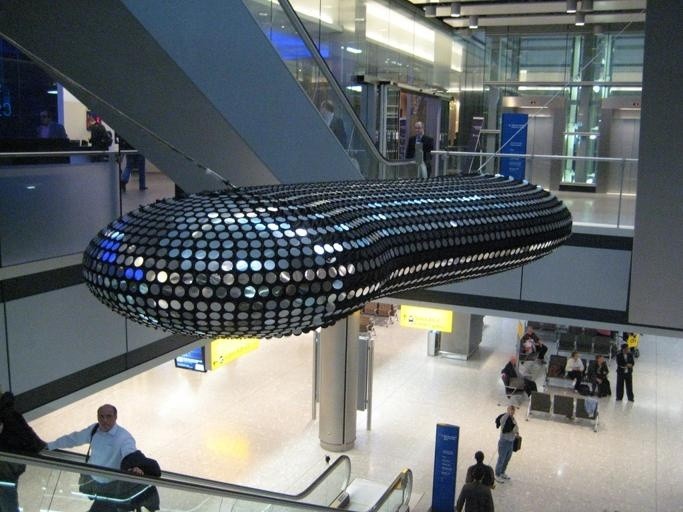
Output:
(428, 330), (441, 356)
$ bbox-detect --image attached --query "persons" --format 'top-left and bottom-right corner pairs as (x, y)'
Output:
(41, 404), (145, 512)
(0, 391), (28, 512)
(586, 355), (609, 398)
(403, 121), (434, 179)
(493, 405), (520, 484)
(564, 352), (585, 394)
(520, 325), (547, 364)
(86, 122), (109, 151)
(319, 100), (345, 149)
(32, 110), (67, 141)
(615, 343), (635, 402)
(465, 451), (495, 490)
(456, 467), (494, 512)
(119, 138), (149, 193)
(500, 356), (536, 396)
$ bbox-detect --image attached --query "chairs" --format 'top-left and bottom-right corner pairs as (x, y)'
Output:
(360, 302), (401, 326)
(499, 321), (618, 432)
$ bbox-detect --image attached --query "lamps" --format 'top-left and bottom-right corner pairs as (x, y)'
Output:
(425, 3), (478, 29)
(566, 0), (593, 26)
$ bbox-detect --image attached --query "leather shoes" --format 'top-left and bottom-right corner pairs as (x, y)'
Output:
(540, 359), (547, 365)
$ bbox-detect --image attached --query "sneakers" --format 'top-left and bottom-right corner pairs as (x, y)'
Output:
(495, 474), (511, 483)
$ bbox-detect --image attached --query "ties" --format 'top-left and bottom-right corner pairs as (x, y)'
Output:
(416, 136), (422, 142)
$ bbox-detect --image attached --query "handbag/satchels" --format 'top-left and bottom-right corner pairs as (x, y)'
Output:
(77, 473), (92, 493)
(579, 384), (590, 395)
